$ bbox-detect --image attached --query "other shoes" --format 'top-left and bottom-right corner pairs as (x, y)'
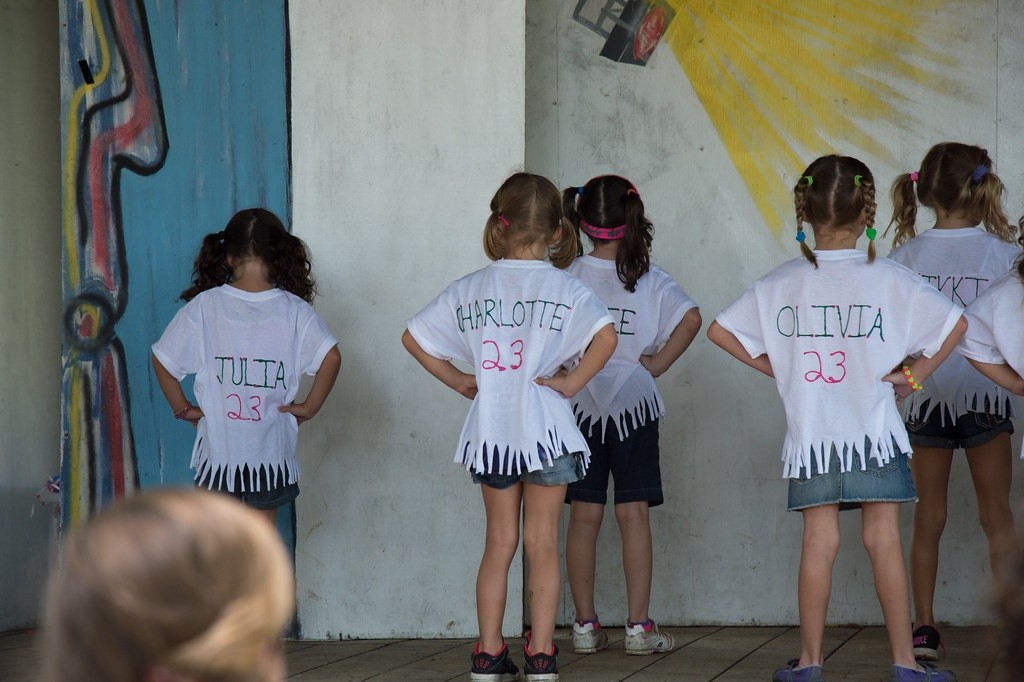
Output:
(773, 658), (825, 682)
(891, 661), (957, 682)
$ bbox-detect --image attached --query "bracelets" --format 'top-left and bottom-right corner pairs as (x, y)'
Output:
(902, 366), (924, 392)
(174, 401), (192, 419)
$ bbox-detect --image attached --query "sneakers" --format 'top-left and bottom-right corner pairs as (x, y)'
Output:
(469, 635), (520, 682)
(523, 631), (559, 682)
(624, 617), (676, 655)
(573, 620), (609, 653)
(911, 622), (940, 659)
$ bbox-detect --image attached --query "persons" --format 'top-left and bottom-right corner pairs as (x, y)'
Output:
(707, 154), (969, 682)
(35, 487), (293, 682)
(562, 174), (702, 656)
(151, 208), (341, 637)
(402, 172), (619, 682)
(952, 211), (1024, 460)
(883, 141), (1024, 660)
